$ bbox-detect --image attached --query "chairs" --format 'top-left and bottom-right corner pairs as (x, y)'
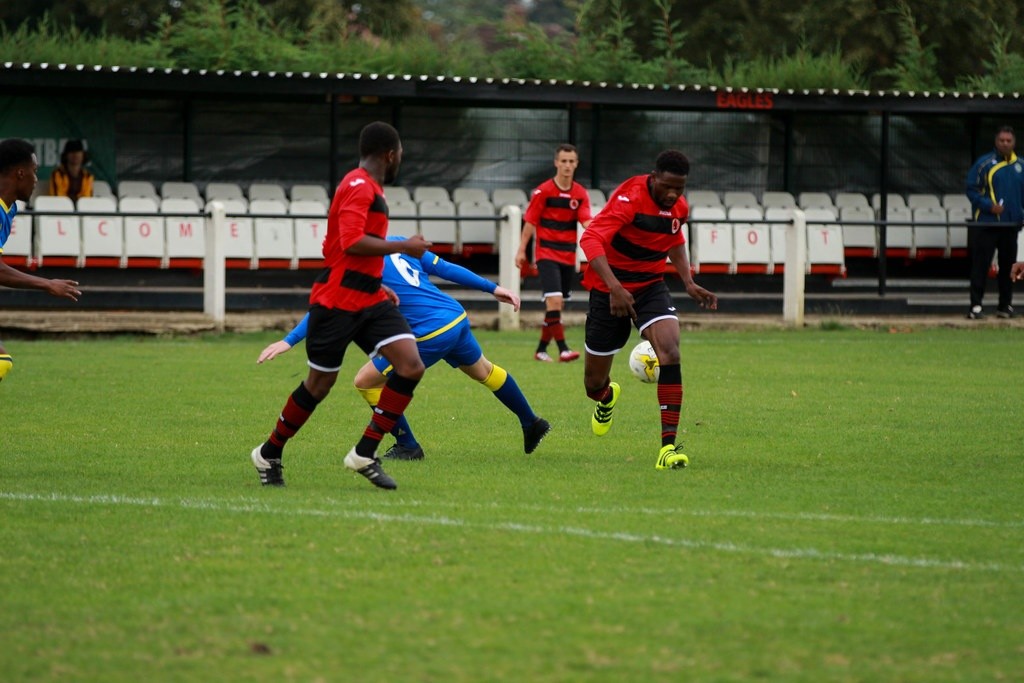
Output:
(0, 181), (973, 290)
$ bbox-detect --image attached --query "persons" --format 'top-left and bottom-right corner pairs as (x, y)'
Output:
(966, 125), (1024, 319)
(50, 138), (93, 207)
(256, 234), (550, 460)
(579, 150), (719, 469)
(249, 121), (434, 489)
(0, 138), (81, 381)
(515, 142), (595, 364)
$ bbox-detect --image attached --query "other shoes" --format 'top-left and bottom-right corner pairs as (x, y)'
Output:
(968, 305), (985, 321)
(995, 305), (1018, 320)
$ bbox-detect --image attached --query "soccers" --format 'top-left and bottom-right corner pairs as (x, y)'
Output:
(628, 340), (661, 383)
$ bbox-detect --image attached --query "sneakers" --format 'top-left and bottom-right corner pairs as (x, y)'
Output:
(522, 417), (551, 454)
(381, 443), (425, 462)
(534, 350), (554, 363)
(592, 383), (620, 435)
(655, 441), (689, 470)
(250, 443), (285, 488)
(342, 447), (397, 490)
(559, 350), (579, 361)
